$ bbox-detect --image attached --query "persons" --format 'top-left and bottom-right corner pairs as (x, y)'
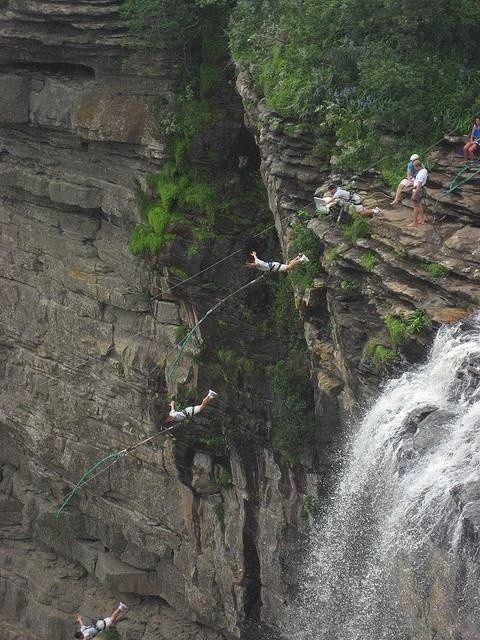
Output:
(389, 154), (428, 226)
(319, 185), (384, 218)
(245, 250), (309, 273)
(164, 390), (217, 426)
(458, 117), (480, 165)
(74, 602), (127, 640)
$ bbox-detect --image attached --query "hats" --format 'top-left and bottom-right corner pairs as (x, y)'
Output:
(410, 154), (418, 160)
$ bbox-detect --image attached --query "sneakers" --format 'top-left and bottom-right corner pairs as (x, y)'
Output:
(119, 602), (127, 609)
(375, 206), (383, 216)
(298, 253), (309, 263)
(208, 389), (217, 396)
